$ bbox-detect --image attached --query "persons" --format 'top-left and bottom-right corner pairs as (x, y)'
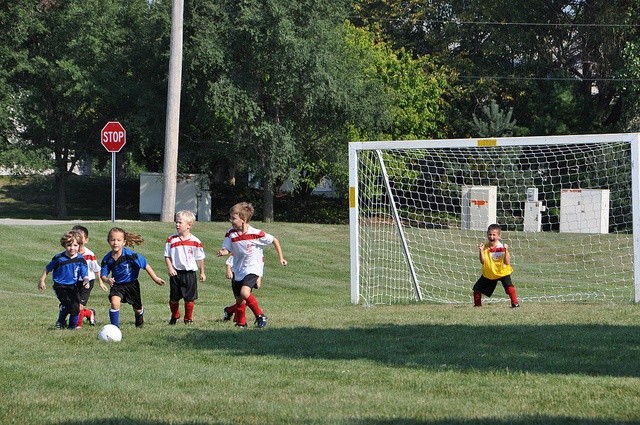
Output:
(164, 210), (205, 327)
(38, 232), (90, 330)
(217, 202), (287, 328)
(100, 227), (166, 330)
(472, 224), (519, 307)
(222, 251), (261, 322)
(70, 226), (108, 329)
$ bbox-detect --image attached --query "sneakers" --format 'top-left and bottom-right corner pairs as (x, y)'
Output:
(169, 316), (176, 325)
(224, 307), (233, 321)
(184, 319), (193, 324)
(135, 314), (143, 328)
(256, 316), (268, 328)
(88, 309), (96, 326)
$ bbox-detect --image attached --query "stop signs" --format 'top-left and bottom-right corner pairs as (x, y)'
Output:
(101, 121), (127, 153)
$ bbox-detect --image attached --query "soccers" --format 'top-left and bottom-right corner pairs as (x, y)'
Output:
(98, 324), (122, 343)
